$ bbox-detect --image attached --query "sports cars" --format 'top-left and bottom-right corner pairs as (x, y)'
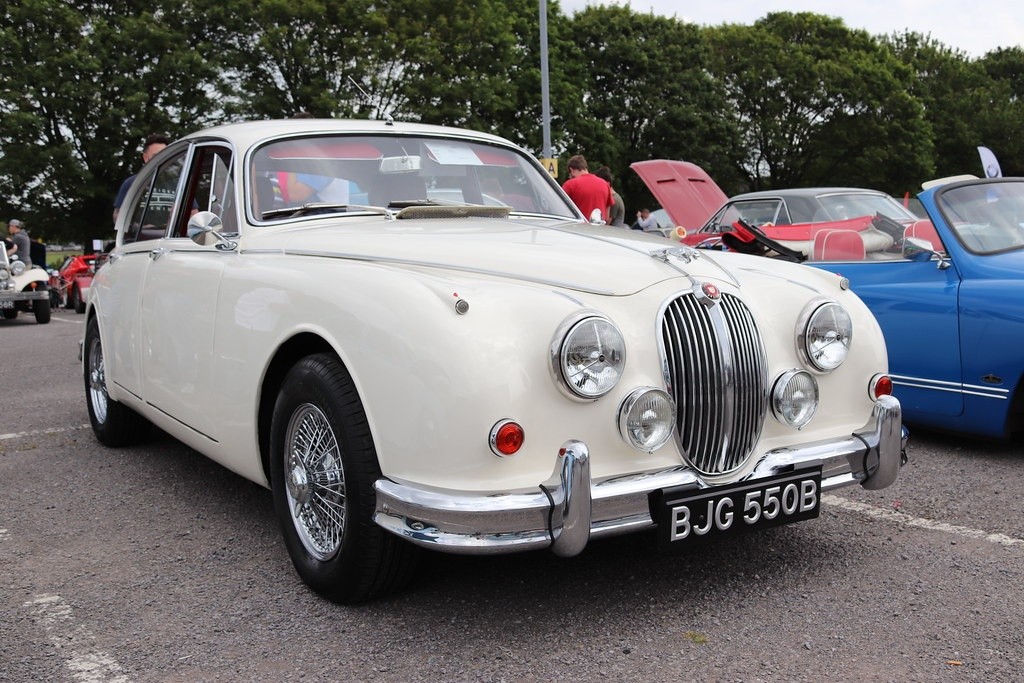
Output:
(800, 177), (1024, 442)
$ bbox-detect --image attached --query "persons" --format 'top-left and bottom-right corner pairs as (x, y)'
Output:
(562, 155), (615, 221)
(29, 231), (46, 270)
(637, 209), (657, 231)
(596, 166), (625, 226)
(482, 179), (536, 212)
(7, 219), (32, 270)
(113, 135), (199, 223)
(286, 172), (349, 208)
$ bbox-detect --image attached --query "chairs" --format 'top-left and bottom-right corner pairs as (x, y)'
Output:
(367, 172), (428, 209)
(813, 227), (866, 262)
(903, 218), (948, 253)
(219, 174), (275, 233)
(156, 178), (210, 230)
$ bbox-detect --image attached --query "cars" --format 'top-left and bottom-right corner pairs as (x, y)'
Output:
(0, 241), (110, 324)
(629, 160), (947, 265)
(79, 119), (908, 597)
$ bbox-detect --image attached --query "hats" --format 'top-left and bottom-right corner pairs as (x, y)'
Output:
(10, 219), (20, 226)
